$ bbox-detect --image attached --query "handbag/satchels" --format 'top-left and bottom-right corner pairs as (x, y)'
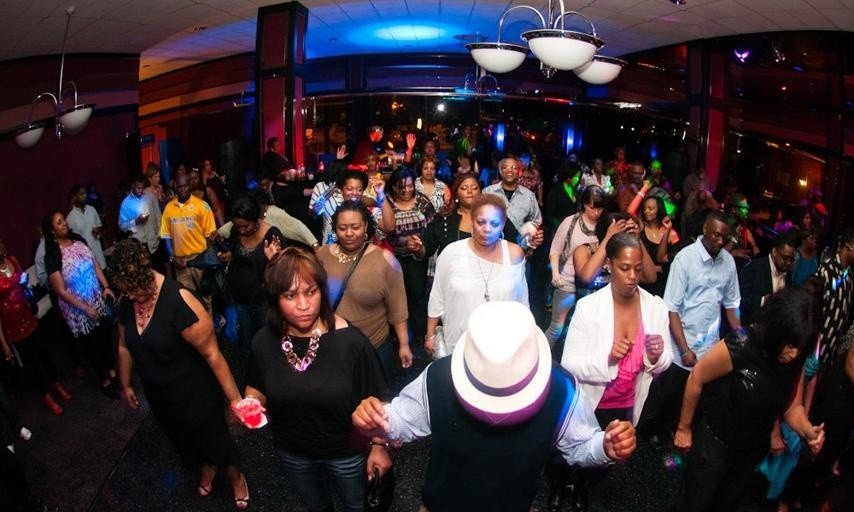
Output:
(365, 462), (395, 512)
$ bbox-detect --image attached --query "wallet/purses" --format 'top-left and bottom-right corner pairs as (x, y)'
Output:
(432, 325), (448, 359)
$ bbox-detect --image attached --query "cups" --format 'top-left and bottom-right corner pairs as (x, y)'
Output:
(235, 398), (262, 427)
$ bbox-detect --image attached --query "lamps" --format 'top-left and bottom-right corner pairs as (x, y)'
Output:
(13, 5), (99, 150)
(463, 1), (628, 85)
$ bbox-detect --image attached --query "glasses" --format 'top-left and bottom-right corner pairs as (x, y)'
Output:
(777, 248), (794, 262)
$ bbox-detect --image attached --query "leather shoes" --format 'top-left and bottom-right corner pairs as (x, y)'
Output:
(198, 465), (218, 496)
(47, 401), (63, 415)
(231, 473), (250, 510)
(61, 390), (71, 400)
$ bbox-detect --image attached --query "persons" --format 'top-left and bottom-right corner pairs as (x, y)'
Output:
(1, 117), (850, 510)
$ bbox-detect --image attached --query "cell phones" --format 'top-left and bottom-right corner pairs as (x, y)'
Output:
(143, 212), (152, 217)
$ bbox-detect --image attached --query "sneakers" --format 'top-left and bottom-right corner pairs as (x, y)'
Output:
(104, 376), (119, 399)
(18, 427), (32, 440)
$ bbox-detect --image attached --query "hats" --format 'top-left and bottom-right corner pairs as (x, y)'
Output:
(449, 300), (553, 424)
